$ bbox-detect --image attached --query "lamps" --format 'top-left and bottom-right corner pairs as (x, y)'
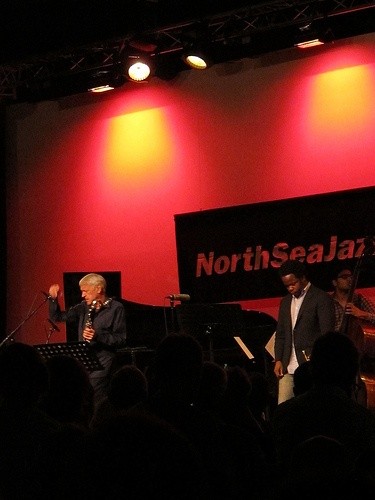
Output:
(181, 37), (214, 73)
(122, 49), (155, 84)
(87, 71), (120, 93)
(294, 23), (325, 49)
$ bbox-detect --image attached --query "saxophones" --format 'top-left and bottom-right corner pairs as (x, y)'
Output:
(83, 298), (113, 343)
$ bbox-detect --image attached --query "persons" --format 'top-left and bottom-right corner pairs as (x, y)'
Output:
(320, 263), (375, 351)
(49, 273), (127, 367)
(272, 261), (334, 404)
(0, 330), (375, 500)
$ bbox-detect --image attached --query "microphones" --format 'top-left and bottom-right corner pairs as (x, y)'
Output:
(40, 290), (56, 303)
(166, 293), (191, 301)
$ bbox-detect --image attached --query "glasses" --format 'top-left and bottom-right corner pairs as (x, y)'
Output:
(336, 274), (353, 280)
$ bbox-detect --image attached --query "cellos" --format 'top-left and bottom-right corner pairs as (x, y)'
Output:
(334, 235), (375, 408)
